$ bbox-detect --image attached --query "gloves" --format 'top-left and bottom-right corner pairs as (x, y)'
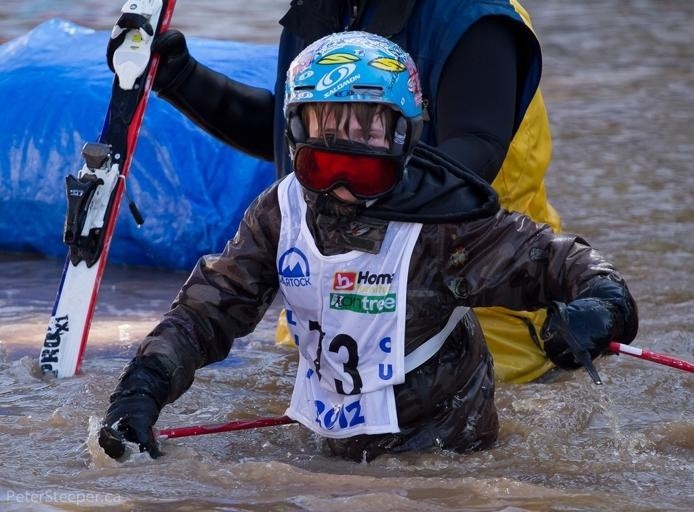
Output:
(107, 13), (189, 92)
(541, 278), (630, 370)
(99, 355), (171, 459)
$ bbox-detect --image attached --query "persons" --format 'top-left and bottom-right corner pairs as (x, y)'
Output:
(106, 0), (561, 383)
(99, 26), (638, 465)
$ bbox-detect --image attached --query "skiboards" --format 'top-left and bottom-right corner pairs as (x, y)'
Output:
(40, 0), (176, 379)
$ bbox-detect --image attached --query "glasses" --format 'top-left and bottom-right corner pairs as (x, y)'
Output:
(293, 143), (407, 199)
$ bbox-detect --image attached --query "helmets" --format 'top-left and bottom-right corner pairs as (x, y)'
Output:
(284, 31), (423, 154)
